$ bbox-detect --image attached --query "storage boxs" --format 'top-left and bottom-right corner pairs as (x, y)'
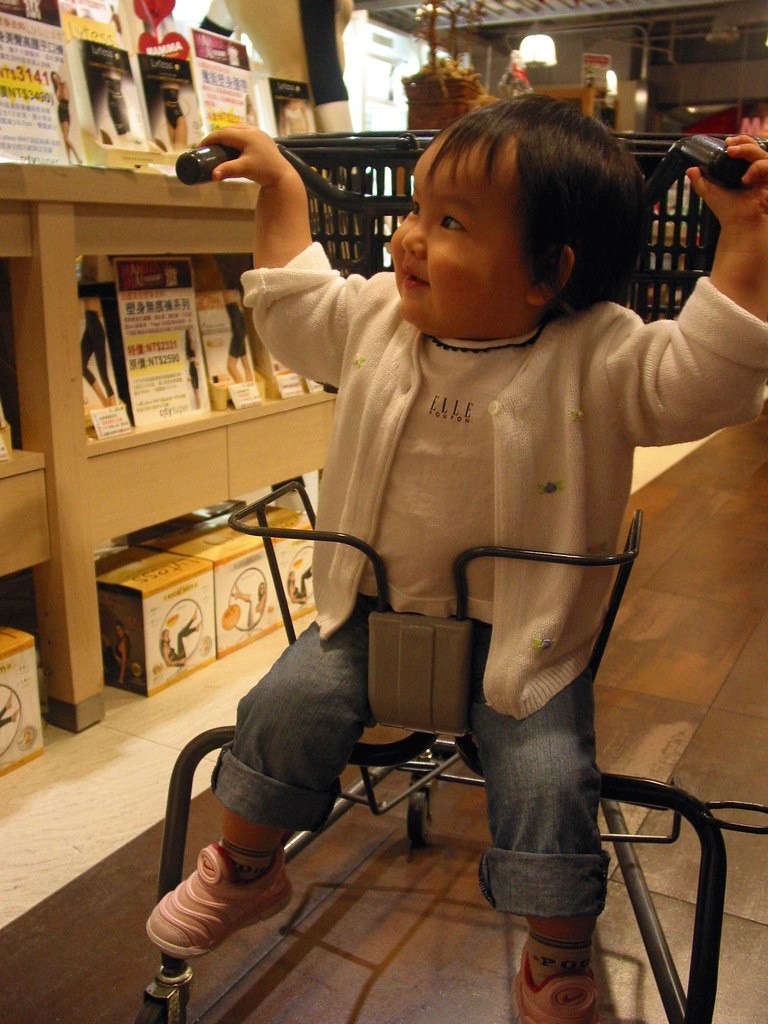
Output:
(0, 497), (317, 773)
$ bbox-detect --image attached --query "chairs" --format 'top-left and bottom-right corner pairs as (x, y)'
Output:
(129, 474), (768, 1024)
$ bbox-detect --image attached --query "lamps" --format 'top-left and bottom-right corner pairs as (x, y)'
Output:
(519, 0), (556, 64)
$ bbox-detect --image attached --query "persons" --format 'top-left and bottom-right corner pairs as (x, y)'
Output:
(112, 620), (131, 685)
(103, 70), (130, 139)
(0, 692), (18, 727)
(232, 582), (266, 628)
(160, 608), (202, 666)
(80, 296), (116, 407)
(50, 71), (82, 165)
(220, 289), (253, 384)
(288, 566), (312, 605)
(148, 92), (768, 1023)
(184, 329), (202, 409)
(156, 80), (187, 153)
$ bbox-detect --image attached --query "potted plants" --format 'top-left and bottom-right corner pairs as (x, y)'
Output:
(401, 0), (487, 132)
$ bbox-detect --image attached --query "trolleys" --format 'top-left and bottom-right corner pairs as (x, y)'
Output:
(132, 130), (768, 1024)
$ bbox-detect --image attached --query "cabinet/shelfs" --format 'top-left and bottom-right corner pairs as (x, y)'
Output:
(0, 160), (338, 732)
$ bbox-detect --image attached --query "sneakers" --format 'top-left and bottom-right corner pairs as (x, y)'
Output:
(146, 842), (293, 958)
(514, 940), (600, 1024)
